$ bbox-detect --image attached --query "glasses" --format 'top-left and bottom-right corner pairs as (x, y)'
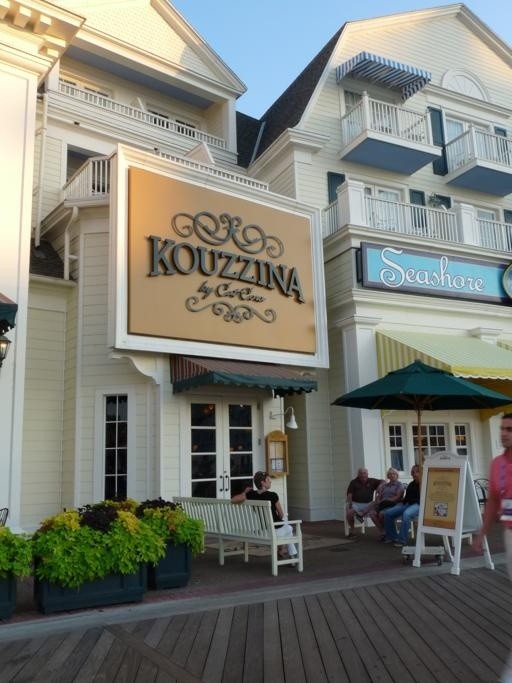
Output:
(257, 472), (270, 478)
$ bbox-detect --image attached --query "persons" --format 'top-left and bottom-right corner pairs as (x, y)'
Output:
(369, 467), (405, 543)
(381, 463), (425, 547)
(469, 412), (512, 683)
(345, 466), (387, 542)
(230, 470), (298, 568)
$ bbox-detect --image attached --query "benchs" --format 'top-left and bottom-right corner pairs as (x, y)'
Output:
(172, 496), (304, 576)
(344, 485), (420, 540)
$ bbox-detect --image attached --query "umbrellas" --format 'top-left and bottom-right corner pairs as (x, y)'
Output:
(328, 357), (512, 481)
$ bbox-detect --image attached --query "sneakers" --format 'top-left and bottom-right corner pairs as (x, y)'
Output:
(377, 534), (407, 547)
(277, 549), (296, 568)
(348, 532), (358, 539)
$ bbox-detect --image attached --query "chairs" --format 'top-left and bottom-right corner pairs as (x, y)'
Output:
(472, 478), (490, 514)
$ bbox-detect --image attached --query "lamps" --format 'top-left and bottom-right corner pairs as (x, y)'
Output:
(271, 405), (297, 430)
